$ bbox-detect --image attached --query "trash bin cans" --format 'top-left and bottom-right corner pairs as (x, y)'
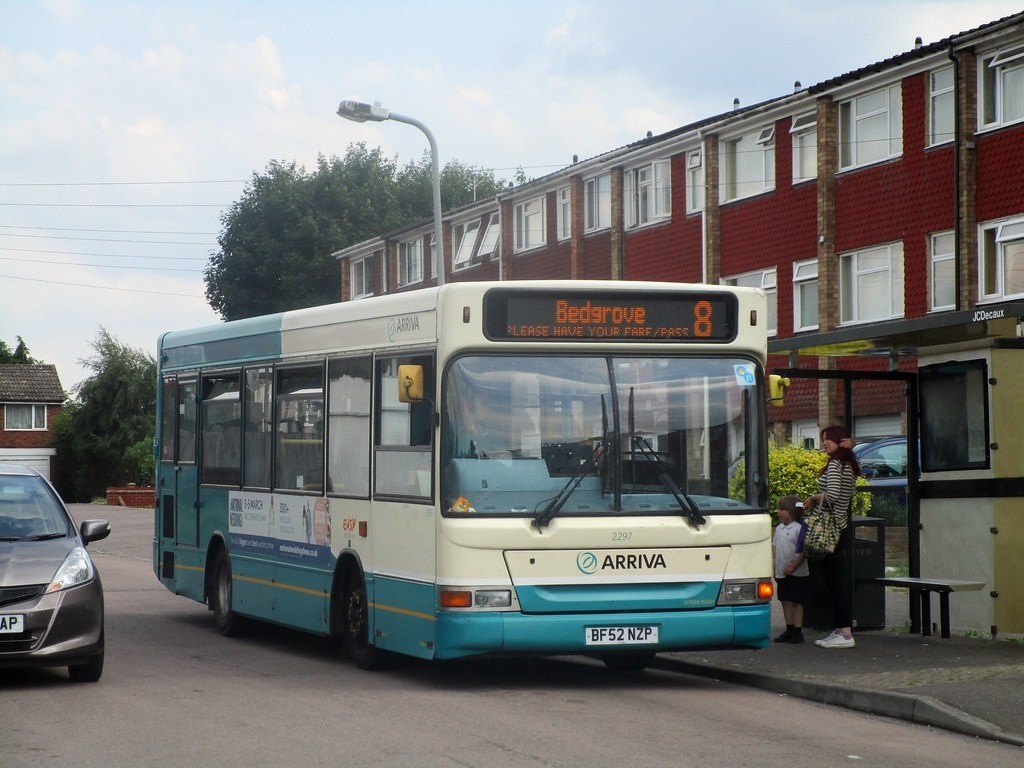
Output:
(801, 517), (885, 633)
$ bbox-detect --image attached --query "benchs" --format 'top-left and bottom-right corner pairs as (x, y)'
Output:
(875, 578), (987, 639)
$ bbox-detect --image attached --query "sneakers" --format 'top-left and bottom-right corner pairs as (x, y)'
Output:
(820, 634), (855, 648)
(814, 628), (841, 645)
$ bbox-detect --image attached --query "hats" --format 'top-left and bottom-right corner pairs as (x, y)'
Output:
(778, 497), (805, 517)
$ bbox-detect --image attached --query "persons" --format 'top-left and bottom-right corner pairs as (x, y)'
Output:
(805, 428), (859, 649)
(776, 496), (808, 644)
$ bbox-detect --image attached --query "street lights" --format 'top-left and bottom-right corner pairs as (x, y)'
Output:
(335, 96), (446, 287)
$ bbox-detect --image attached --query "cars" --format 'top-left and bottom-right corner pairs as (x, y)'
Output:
(850, 437), (926, 526)
(1, 463), (111, 682)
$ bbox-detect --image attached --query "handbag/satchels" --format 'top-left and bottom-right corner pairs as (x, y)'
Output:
(795, 511), (814, 553)
(803, 492), (841, 553)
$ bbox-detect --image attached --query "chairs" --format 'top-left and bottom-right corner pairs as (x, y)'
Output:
(541, 412), (598, 477)
(164, 419), (324, 489)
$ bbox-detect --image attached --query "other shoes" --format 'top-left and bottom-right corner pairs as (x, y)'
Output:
(774, 624), (794, 642)
(790, 627), (804, 644)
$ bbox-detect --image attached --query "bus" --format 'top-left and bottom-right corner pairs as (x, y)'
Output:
(152, 279), (791, 677)
